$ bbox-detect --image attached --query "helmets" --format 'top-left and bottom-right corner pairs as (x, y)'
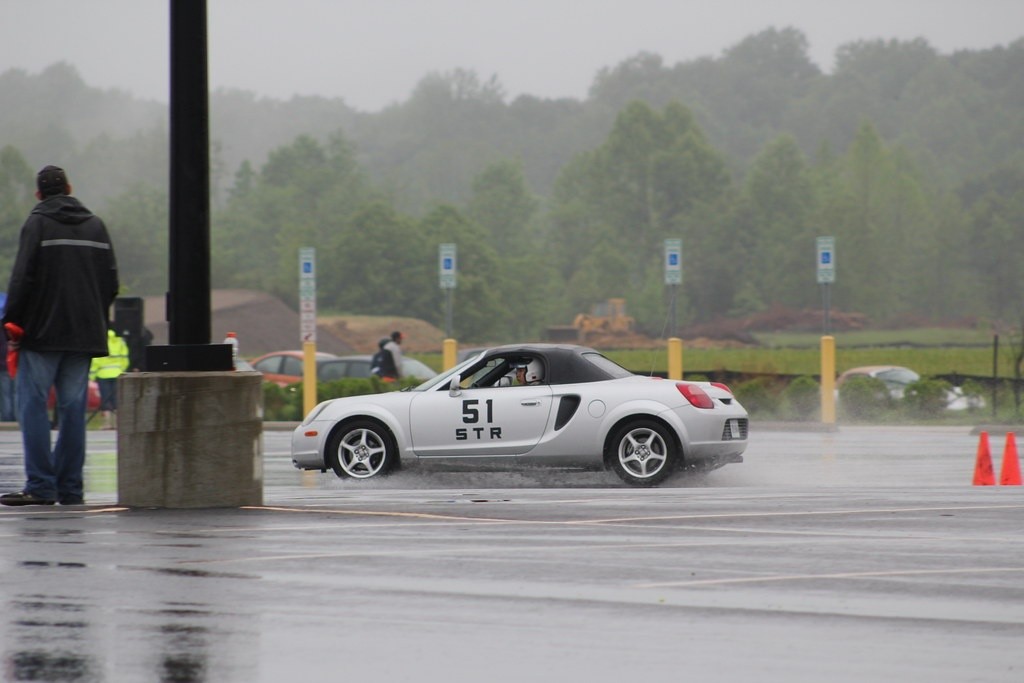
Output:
(510, 356), (544, 382)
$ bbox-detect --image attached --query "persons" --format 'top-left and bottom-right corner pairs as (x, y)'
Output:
(0, 165), (119, 505)
(89, 329), (130, 431)
(509, 355), (545, 385)
(371, 331), (405, 383)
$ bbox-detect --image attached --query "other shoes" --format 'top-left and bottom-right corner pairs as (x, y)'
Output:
(2, 490), (55, 504)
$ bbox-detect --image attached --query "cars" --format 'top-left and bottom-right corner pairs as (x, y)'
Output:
(250, 350), (437, 409)
(47, 380), (101, 413)
(833, 366), (987, 414)
(291, 343), (749, 488)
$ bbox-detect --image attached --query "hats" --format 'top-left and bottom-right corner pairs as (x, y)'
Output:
(37, 165), (66, 192)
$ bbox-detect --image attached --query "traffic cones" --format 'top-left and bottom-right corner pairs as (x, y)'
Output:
(999, 432), (1024, 485)
(972, 431), (997, 485)
